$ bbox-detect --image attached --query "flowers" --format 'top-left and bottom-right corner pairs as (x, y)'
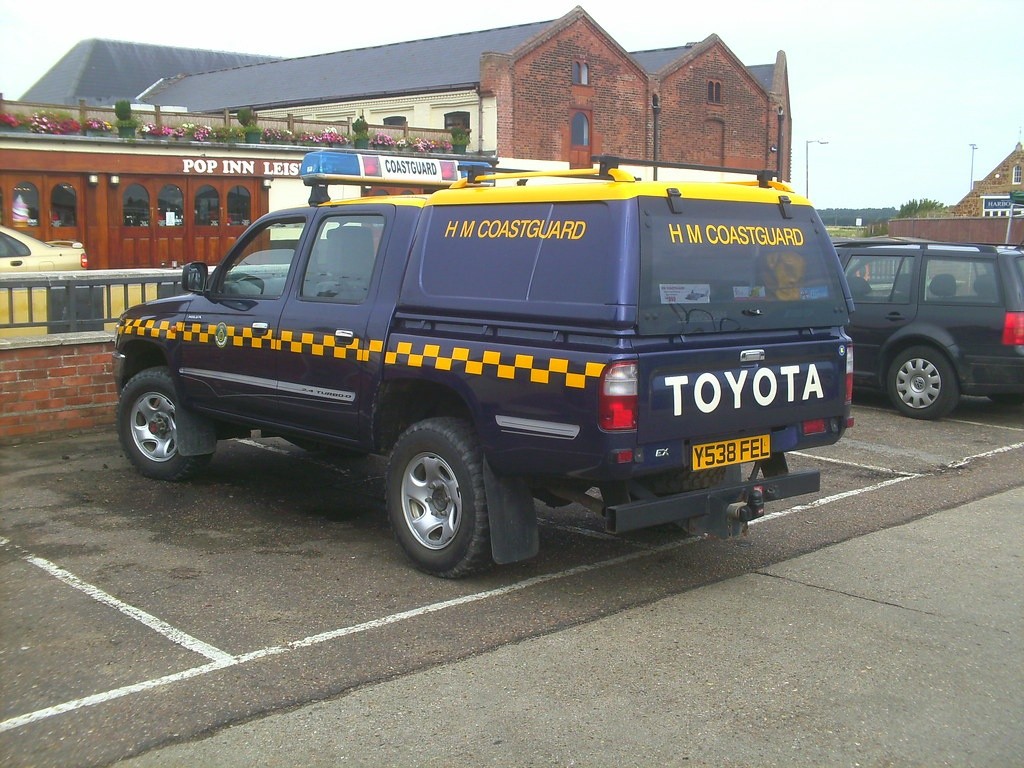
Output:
(440, 140), (452, 150)
(136, 123), (172, 135)
(295, 130), (331, 144)
(329, 131), (355, 145)
(80, 116), (114, 133)
(397, 138), (430, 151)
(171, 123), (199, 136)
(0, 112), (31, 133)
(368, 131), (396, 147)
(263, 128), (294, 143)
(428, 139), (443, 149)
(193, 125), (245, 142)
(27, 110), (80, 135)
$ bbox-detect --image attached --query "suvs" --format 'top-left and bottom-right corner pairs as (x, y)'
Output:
(833, 238), (1023, 421)
(111, 148), (860, 582)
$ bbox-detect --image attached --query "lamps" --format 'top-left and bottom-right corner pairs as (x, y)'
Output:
(263, 177), (274, 188)
(771, 144), (777, 152)
(88, 173), (99, 186)
(110, 173), (120, 186)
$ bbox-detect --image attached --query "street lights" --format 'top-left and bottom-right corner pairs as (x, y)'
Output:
(968, 143), (978, 191)
(805, 138), (830, 200)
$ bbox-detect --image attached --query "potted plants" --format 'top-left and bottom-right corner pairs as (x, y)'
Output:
(352, 115), (370, 149)
(114, 100), (140, 138)
(450, 125), (472, 154)
(237, 106), (262, 144)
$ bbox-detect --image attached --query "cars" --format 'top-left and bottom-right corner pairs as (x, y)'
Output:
(0, 223), (89, 274)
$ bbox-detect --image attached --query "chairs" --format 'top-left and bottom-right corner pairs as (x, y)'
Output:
(928, 274), (958, 300)
(315, 225), (374, 300)
(972, 274), (996, 303)
(893, 274), (910, 303)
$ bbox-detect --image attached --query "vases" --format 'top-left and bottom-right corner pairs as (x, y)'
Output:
(330, 142), (353, 148)
(143, 135), (167, 140)
(175, 135), (196, 141)
(431, 148), (441, 153)
(86, 129), (113, 137)
(374, 145), (393, 150)
(399, 147), (412, 151)
